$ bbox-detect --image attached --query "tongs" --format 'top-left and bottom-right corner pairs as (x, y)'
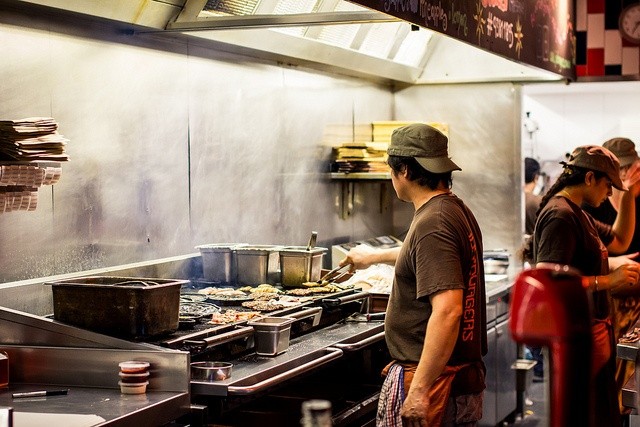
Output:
(317, 261), (356, 288)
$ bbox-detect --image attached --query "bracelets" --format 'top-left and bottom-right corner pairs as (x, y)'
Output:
(595, 275), (599, 291)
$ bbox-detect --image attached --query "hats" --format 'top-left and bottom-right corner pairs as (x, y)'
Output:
(559, 146), (630, 192)
(388, 123), (462, 173)
(602, 137), (638, 167)
(525, 158), (548, 176)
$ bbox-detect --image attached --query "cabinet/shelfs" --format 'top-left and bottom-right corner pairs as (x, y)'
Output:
(483, 290), (522, 427)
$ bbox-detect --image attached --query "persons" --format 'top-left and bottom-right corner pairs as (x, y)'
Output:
(523, 157), (550, 234)
(581, 137), (639, 427)
(533, 145), (639, 427)
(339, 123), (488, 426)
